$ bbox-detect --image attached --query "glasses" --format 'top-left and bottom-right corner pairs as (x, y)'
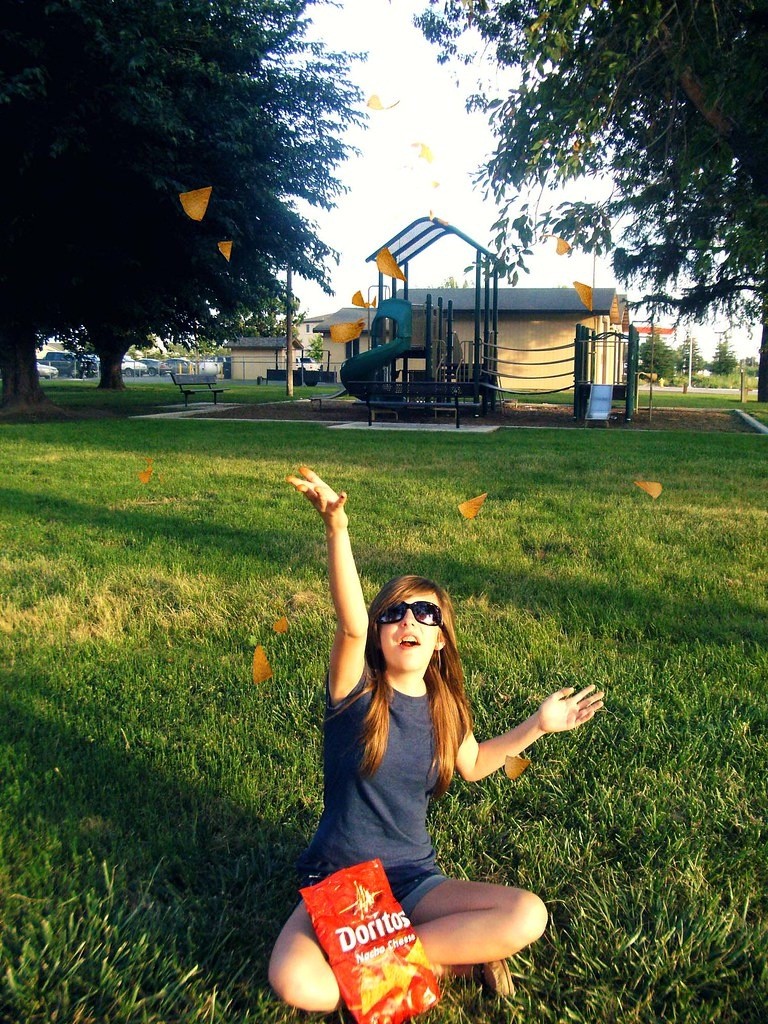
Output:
(372, 597), (448, 631)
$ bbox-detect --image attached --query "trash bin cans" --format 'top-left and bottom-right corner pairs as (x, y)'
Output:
(87, 362), (99, 378)
(257, 376), (263, 385)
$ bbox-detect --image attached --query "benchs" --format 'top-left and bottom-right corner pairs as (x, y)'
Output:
(170, 372), (230, 407)
(348, 381), (483, 429)
(265, 369), (304, 386)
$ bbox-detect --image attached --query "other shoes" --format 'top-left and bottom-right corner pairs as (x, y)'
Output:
(480, 956), (515, 1001)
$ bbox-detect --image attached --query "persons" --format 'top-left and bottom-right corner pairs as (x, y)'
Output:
(268, 466), (604, 1013)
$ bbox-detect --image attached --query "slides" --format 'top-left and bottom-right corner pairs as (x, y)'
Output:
(340, 338), (411, 401)
(583, 384), (614, 420)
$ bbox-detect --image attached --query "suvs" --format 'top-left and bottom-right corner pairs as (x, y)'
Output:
(45, 351), (93, 378)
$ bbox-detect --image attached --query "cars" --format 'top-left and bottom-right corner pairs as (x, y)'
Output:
(82, 350), (231, 384)
(36, 361), (59, 379)
